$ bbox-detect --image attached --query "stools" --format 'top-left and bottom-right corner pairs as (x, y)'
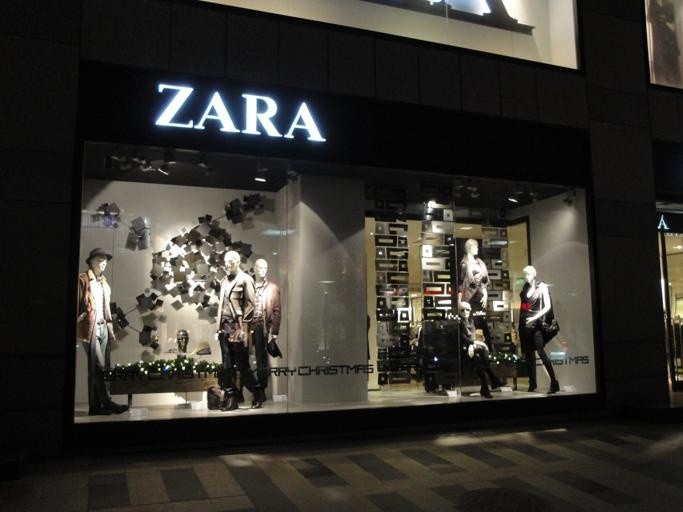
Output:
(97, 320), (104, 325)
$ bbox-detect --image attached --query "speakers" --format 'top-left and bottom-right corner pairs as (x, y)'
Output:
(547, 380), (560, 394)
(527, 381), (537, 392)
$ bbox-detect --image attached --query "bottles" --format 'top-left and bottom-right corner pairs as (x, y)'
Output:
(86, 248), (112, 264)
(268, 338), (282, 359)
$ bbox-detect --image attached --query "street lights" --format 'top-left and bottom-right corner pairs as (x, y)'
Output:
(208, 386), (227, 410)
(543, 319), (559, 342)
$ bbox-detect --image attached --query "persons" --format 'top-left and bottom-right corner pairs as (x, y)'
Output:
(451, 300), (507, 399)
(517, 264), (560, 395)
(213, 258), (281, 403)
(216, 250), (269, 411)
(458, 238), (493, 353)
(77, 246), (129, 416)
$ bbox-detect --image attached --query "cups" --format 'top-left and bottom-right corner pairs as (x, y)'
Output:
(460, 308), (470, 312)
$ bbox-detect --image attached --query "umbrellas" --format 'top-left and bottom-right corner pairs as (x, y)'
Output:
(481, 390), (493, 398)
(492, 381), (507, 389)
(88, 405), (112, 415)
(220, 393), (263, 410)
(104, 402), (129, 414)
